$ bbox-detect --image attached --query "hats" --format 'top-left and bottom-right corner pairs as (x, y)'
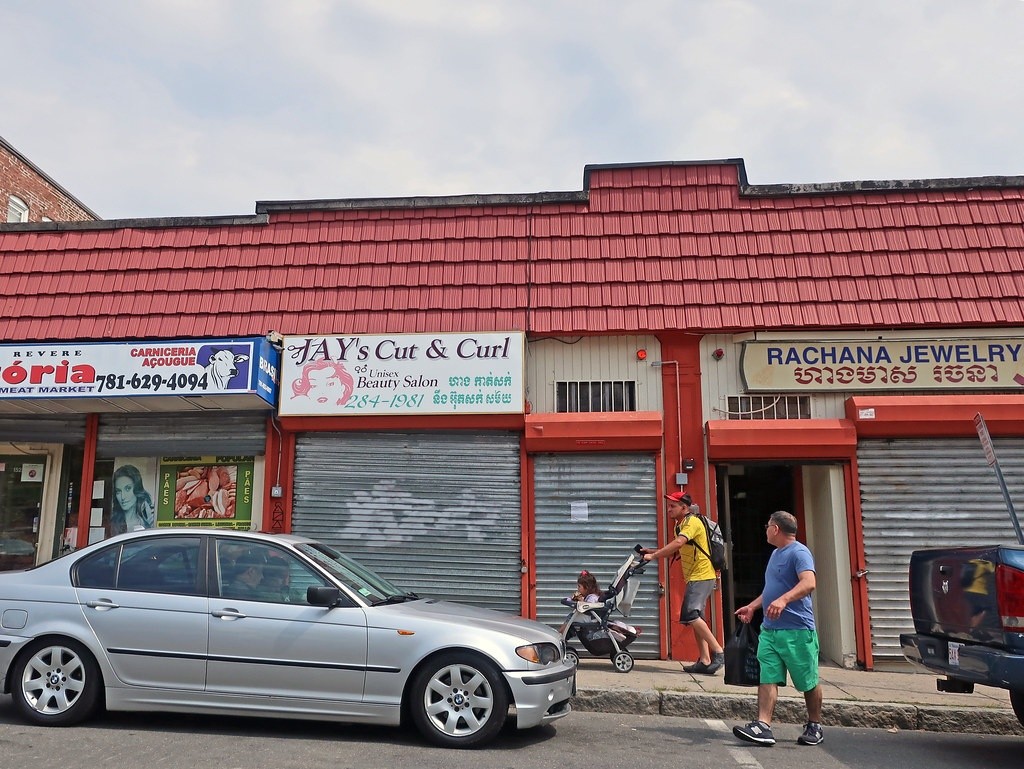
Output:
(664, 491), (692, 506)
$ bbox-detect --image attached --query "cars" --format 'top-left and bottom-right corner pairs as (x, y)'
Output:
(1, 527), (576, 746)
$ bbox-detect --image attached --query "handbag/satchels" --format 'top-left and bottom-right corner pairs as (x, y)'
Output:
(724, 620), (787, 687)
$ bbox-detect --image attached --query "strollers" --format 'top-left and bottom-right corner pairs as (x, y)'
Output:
(555, 554), (651, 675)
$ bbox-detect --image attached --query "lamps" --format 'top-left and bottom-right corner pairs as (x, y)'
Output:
(713, 348), (724, 359)
(637, 348), (647, 360)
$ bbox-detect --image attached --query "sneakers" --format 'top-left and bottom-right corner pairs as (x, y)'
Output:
(732, 722), (776, 744)
(683, 657), (715, 674)
(706, 651), (725, 674)
(797, 725), (824, 745)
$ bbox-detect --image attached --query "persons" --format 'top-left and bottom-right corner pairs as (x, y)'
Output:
(225, 555), (265, 600)
(558, 570), (602, 634)
(639, 492), (725, 675)
(255, 563), (287, 602)
(732, 511), (824, 746)
(109, 465), (155, 535)
(960, 558), (996, 638)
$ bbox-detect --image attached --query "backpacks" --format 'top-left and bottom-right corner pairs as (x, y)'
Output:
(676, 512), (726, 572)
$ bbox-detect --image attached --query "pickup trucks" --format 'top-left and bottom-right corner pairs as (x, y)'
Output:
(899, 542), (1024, 721)
